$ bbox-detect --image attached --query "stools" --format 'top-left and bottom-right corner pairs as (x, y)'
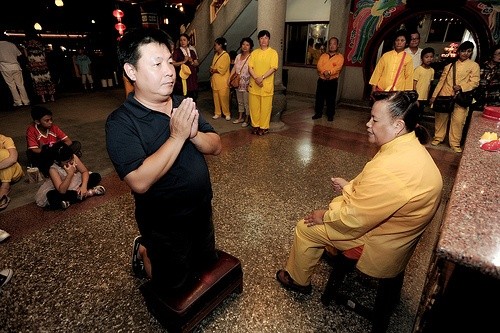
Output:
(321, 250), (405, 333)
(141, 249), (243, 333)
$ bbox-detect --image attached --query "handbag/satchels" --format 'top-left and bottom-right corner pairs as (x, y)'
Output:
(433, 96), (455, 113)
(455, 91), (472, 108)
(231, 74), (240, 87)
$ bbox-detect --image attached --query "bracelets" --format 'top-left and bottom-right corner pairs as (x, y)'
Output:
(190, 61), (194, 65)
(262, 75), (265, 79)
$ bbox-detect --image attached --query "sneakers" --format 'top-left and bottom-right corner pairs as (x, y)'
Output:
(89, 185), (106, 196)
(0, 229), (11, 242)
(131, 235), (147, 275)
(61, 200), (70, 208)
(0, 194), (11, 210)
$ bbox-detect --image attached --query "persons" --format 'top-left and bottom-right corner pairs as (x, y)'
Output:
(0, 229), (11, 242)
(0, 28), (122, 109)
(211, 30), (278, 136)
(35, 141), (106, 208)
(312, 37), (344, 122)
(170, 34), (200, 100)
(24, 106), (80, 179)
(276, 90), (444, 294)
(0, 134), (26, 208)
(0, 268), (13, 288)
(106, 27), (222, 299)
(369, 29), (500, 152)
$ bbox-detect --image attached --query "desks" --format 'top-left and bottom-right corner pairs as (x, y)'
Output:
(412, 110), (500, 333)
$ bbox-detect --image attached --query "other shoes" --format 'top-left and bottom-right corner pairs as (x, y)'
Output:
(277, 270), (311, 294)
(312, 113), (322, 119)
(258, 130), (269, 135)
(450, 147), (462, 152)
(226, 116), (231, 120)
(0, 268), (13, 287)
(233, 119), (245, 124)
(212, 114), (222, 118)
(252, 128), (258, 134)
(328, 115), (334, 121)
(431, 140), (441, 145)
(241, 122), (248, 127)
(12, 103), (29, 111)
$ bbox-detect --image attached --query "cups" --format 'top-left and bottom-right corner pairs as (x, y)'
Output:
(27, 168), (39, 183)
